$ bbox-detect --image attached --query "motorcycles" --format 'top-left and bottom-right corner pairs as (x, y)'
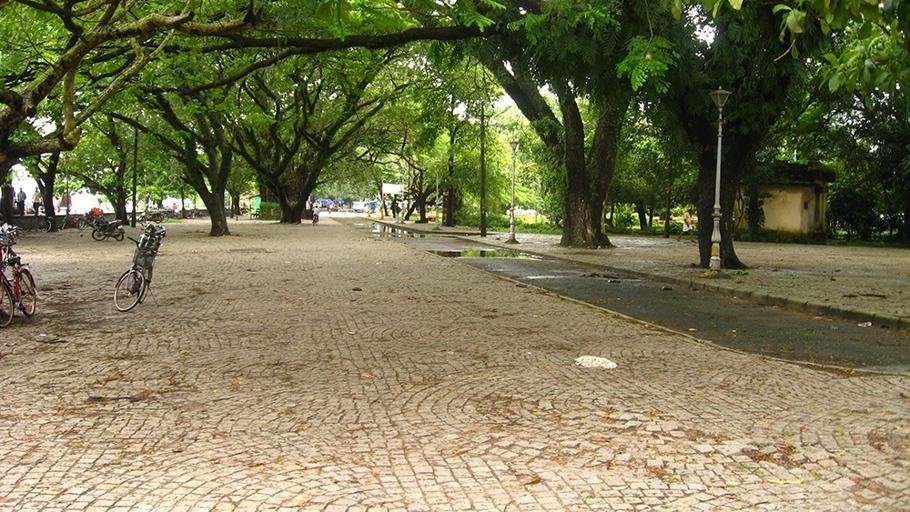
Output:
(138, 209), (169, 224)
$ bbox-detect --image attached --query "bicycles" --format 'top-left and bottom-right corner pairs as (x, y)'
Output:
(0, 223), (37, 328)
(60, 208), (125, 241)
(23, 210), (52, 232)
(312, 215), (318, 226)
(114, 222), (166, 312)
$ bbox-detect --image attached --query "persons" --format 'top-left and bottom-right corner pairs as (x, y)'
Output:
(32, 187), (41, 215)
(172, 203), (177, 209)
(677, 209), (697, 243)
(312, 206), (320, 221)
(11, 187), (15, 205)
(17, 187), (26, 216)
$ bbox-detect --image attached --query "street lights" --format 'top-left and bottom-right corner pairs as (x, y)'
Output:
(708, 86), (731, 271)
(505, 136), (519, 244)
